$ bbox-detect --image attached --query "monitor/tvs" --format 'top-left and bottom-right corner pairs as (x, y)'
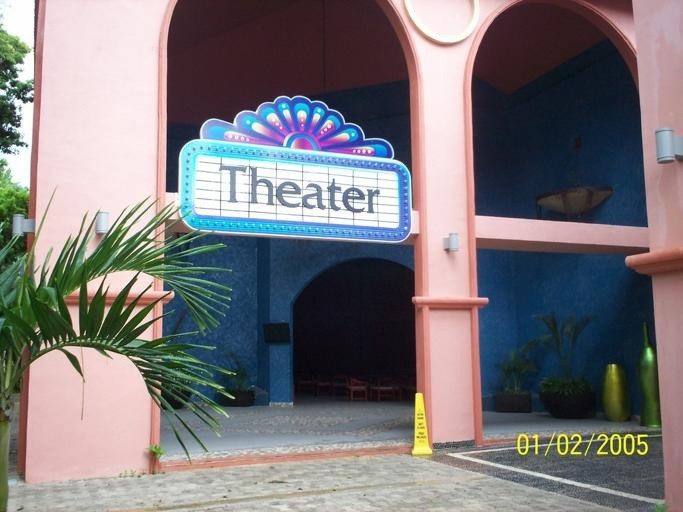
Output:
(264, 323), (290, 343)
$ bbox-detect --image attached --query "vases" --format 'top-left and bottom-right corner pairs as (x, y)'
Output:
(603, 362), (633, 422)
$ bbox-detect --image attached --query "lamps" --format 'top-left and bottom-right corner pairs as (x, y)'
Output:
(443, 232), (459, 253)
(654, 128), (682, 163)
(11, 214), (35, 236)
(96, 211), (108, 235)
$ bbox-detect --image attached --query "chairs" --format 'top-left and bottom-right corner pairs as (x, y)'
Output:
(293, 371), (416, 401)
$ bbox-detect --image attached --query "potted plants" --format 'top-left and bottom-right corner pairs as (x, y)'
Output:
(216, 352), (255, 407)
(161, 351), (194, 410)
(494, 311), (599, 418)
